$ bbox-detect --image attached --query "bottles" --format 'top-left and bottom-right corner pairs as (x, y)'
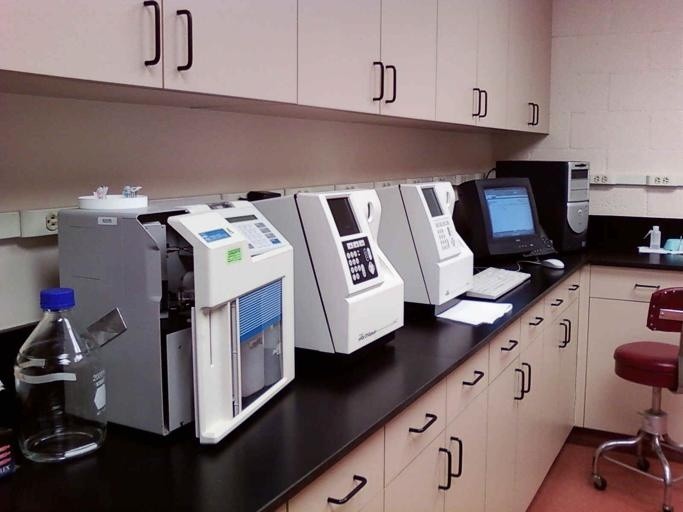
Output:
(649, 253), (660, 264)
(13, 285), (109, 464)
(649, 225), (662, 251)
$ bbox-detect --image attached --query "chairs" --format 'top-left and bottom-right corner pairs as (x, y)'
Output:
(590, 287), (681, 511)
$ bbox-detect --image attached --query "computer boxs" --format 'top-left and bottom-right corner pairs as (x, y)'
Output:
(495, 159), (590, 253)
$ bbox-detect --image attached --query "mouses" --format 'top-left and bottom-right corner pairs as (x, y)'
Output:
(542, 258), (564, 269)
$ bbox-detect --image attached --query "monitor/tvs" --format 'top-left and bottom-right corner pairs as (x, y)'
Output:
(457, 177), (556, 256)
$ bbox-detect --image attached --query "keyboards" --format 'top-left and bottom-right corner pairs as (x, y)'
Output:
(467, 266), (532, 299)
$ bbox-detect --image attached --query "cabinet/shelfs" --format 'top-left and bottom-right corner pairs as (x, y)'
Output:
(277, 424), (385, 512)
(0, 0), (298, 109)
(485, 298), (545, 512)
(543, 270), (578, 478)
(396, 3), (550, 136)
(582, 264), (681, 451)
(188, 1), (436, 126)
(385, 340), (490, 511)
